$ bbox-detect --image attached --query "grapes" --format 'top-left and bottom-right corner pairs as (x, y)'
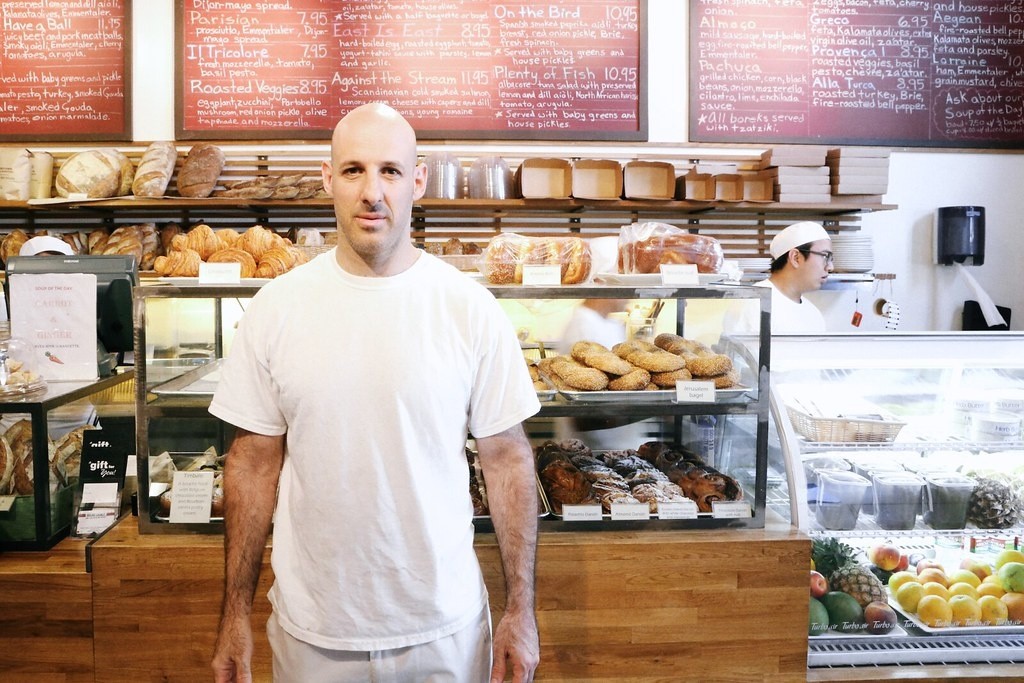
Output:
(810, 537), (888, 610)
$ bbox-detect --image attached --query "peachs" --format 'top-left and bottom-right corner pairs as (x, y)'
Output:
(865, 601), (897, 634)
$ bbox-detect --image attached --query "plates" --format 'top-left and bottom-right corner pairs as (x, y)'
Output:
(724, 257), (772, 282)
(827, 234), (874, 273)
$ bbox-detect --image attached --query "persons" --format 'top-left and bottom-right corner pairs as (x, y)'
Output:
(0, 236), (103, 442)
(718, 219), (834, 470)
(554, 235), (647, 451)
(209, 101), (541, 683)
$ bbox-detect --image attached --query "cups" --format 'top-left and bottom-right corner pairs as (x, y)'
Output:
(624, 315), (658, 346)
(800, 450), (978, 529)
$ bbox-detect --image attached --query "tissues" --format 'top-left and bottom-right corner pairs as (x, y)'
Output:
(932, 205), (1008, 327)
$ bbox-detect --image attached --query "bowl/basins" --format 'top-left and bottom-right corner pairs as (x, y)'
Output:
(422, 152), (464, 200)
(468, 156), (514, 199)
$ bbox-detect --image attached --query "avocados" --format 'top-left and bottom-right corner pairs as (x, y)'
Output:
(808, 590), (863, 635)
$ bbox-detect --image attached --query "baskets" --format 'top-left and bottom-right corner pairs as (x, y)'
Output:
(785, 401), (906, 447)
(76, 378), (135, 405)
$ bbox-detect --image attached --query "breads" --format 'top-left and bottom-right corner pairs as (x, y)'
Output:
(412, 232), (723, 284)
(530, 332), (742, 394)
(464, 437), (733, 516)
(0, 140), (338, 278)
(160, 475), (226, 518)
(0, 359), (97, 499)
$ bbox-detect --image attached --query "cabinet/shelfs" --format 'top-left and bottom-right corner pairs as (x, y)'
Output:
(0, 142), (902, 535)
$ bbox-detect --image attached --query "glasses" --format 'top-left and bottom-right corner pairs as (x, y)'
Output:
(799, 249), (834, 261)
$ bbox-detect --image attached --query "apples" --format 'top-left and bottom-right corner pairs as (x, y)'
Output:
(809, 571), (827, 597)
(870, 543), (1024, 626)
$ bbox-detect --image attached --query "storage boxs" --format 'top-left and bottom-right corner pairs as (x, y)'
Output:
(674, 174), (715, 203)
(622, 158), (676, 200)
(572, 160), (623, 201)
(744, 173), (773, 204)
(515, 157), (572, 198)
(713, 174), (744, 202)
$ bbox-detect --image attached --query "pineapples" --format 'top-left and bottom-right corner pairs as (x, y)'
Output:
(953, 464), (1021, 530)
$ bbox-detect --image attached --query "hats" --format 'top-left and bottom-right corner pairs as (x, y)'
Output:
(18, 235), (74, 256)
(769, 222), (831, 261)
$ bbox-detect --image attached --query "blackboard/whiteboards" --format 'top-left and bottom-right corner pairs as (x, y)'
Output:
(688, 1), (1024, 153)
(173, 2), (653, 145)
(1, 1), (138, 144)
